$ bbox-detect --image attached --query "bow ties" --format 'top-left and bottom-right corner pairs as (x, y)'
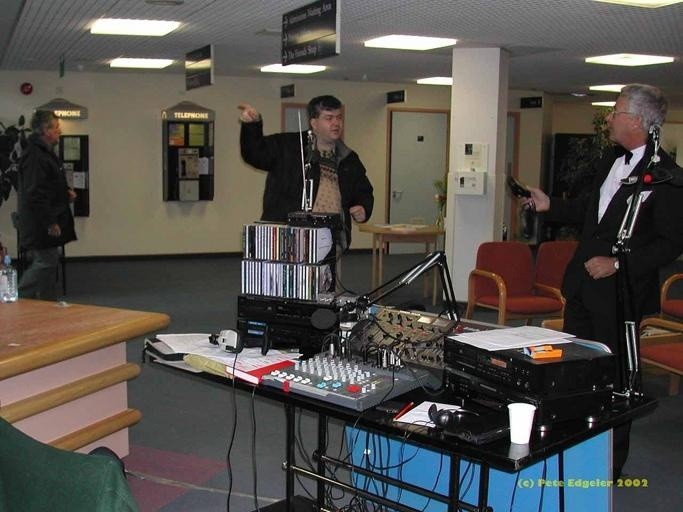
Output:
(613, 143), (633, 164)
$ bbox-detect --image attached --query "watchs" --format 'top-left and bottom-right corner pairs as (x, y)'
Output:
(614, 259), (619, 274)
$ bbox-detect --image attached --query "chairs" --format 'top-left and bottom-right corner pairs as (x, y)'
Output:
(660, 274), (683, 317)
(536, 239), (578, 286)
(465, 243), (565, 324)
(638, 316), (683, 379)
(0, 417), (318, 512)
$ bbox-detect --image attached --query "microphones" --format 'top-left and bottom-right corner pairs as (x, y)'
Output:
(311, 308), (349, 329)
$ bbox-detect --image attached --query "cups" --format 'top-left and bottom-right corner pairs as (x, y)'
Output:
(508, 442), (530, 460)
(507, 403), (536, 445)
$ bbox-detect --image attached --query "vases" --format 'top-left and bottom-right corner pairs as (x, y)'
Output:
(433, 195), (446, 230)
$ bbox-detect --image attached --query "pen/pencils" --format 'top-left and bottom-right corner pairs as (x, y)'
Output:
(392, 402), (414, 421)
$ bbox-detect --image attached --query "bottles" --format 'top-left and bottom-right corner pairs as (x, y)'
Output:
(0, 255), (18, 304)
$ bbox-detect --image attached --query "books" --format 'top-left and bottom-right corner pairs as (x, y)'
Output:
(391, 226), (416, 232)
(242, 223), (333, 264)
(225, 359), (295, 384)
(241, 260), (331, 301)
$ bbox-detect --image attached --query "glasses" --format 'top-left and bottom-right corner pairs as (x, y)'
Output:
(610, 107), (633, 119)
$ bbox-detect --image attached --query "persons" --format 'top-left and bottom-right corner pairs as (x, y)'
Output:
(239, 94), (373, 292)
(520, 84), (683, 482)
(16, 110), (76, 299)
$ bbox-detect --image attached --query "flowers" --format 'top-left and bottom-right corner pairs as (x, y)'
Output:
(433, 181), (447, 225)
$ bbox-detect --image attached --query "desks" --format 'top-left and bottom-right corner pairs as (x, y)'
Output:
(360, 225), (445, 307)
(142, 338), (658, 512)
(1, 298), (171, 459)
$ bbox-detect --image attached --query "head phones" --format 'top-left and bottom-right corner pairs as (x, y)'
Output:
(209, 325), (273, 355)
(429, 399), (487, 427)
